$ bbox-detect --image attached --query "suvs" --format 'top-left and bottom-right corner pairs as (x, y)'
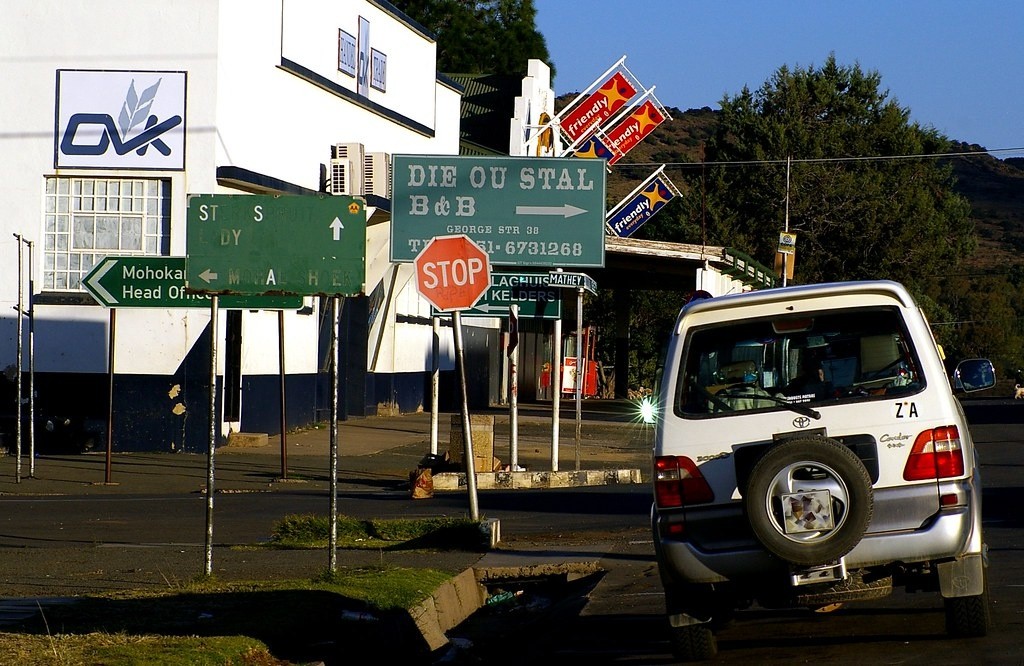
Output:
(645, 275), (991, 661)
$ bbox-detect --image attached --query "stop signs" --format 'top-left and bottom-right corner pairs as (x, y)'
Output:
(414, 235), (492, 314)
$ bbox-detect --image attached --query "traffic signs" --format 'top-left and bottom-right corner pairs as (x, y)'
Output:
(429, 273), (562, 318)
(82, 254), (305, 311)
(389, 152), (606, 268)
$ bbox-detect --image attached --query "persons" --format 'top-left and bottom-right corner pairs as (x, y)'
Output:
(933, 329), (946, 361)
(0, 366), (40, 457)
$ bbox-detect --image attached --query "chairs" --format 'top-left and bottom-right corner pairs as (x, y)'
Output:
(706, 347), (766, 411)
(853, 335), (911, 392)
